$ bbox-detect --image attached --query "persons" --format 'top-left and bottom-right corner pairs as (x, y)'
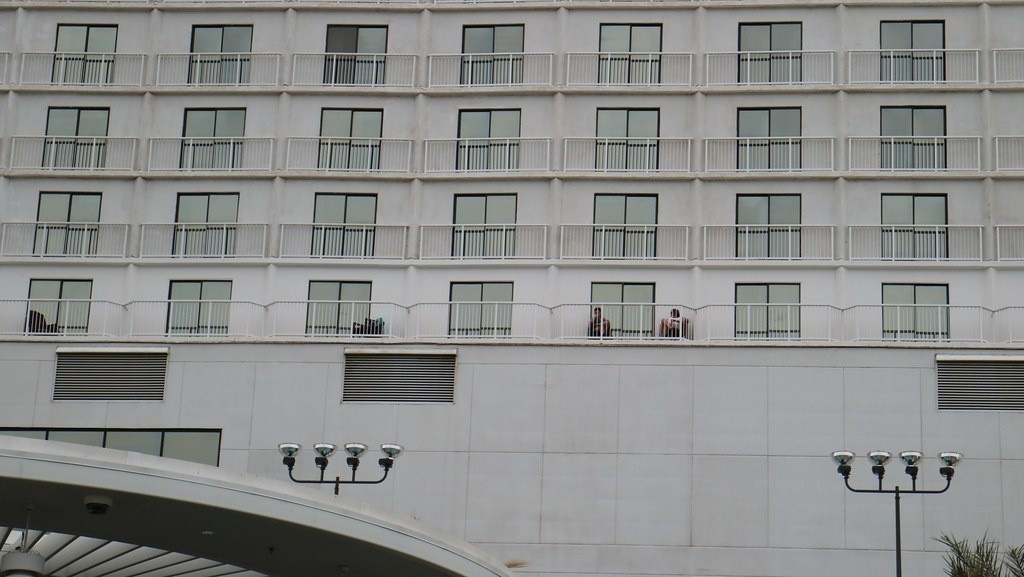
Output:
(587, 308), (612, 337)
(661, 308), (689, 338)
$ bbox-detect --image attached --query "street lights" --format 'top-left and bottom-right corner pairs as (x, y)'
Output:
(830, 450), (965, 577)
(277, 442), (404, 499)
